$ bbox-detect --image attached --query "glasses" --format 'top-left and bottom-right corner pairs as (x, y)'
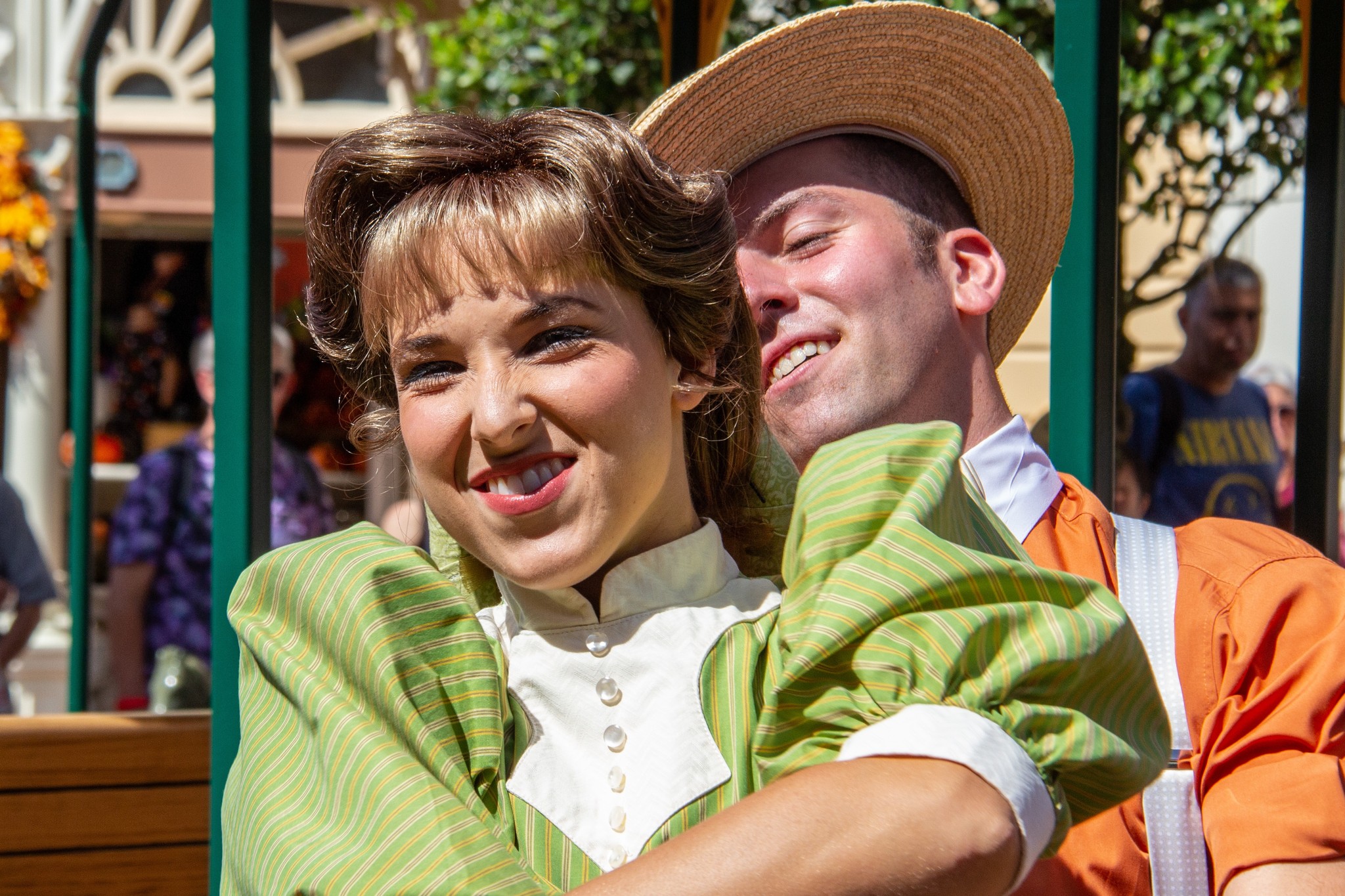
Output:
(270, 370), (285, 387)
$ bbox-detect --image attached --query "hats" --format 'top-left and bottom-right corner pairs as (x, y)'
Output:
(626, 0), (1073, 372)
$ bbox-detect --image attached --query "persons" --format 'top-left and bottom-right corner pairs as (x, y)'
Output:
(108, 242), (202, 465)
(0, 477), (59, 715)
(217, 107), (1172, 896)
(103, 319), (342, 716)
(618, 1), (1345, 896)
(1114, 253), (1297, 530)
